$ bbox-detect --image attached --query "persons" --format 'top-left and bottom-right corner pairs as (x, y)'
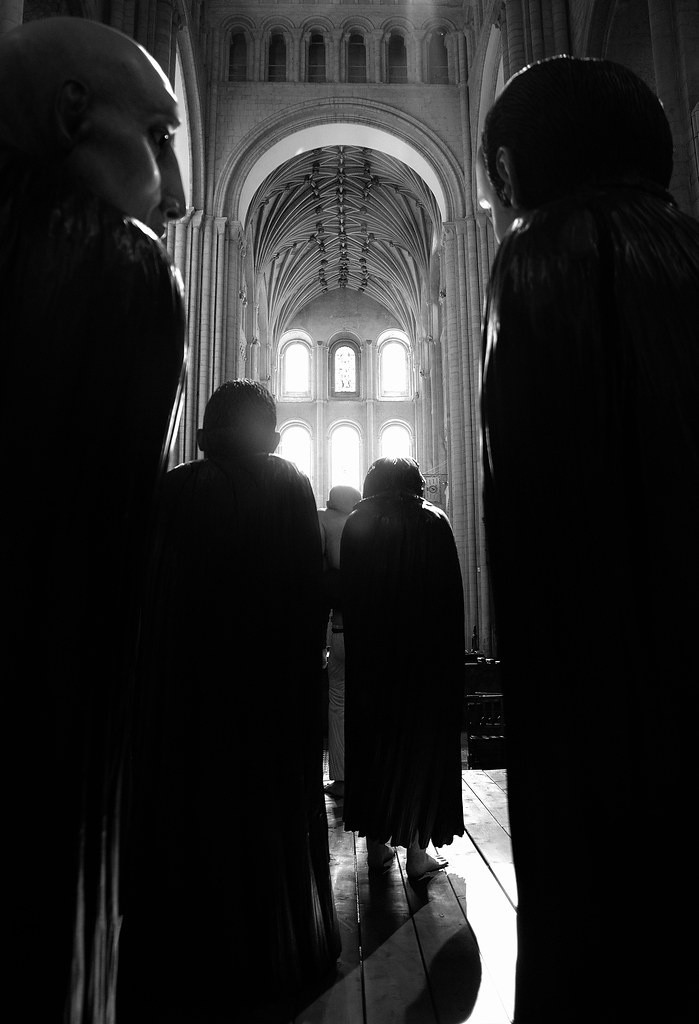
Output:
(472, 54), (699, 1024)
(1, 16), (468, 1024)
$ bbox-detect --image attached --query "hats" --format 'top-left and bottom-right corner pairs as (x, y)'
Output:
(326, 486), (361, 512)
